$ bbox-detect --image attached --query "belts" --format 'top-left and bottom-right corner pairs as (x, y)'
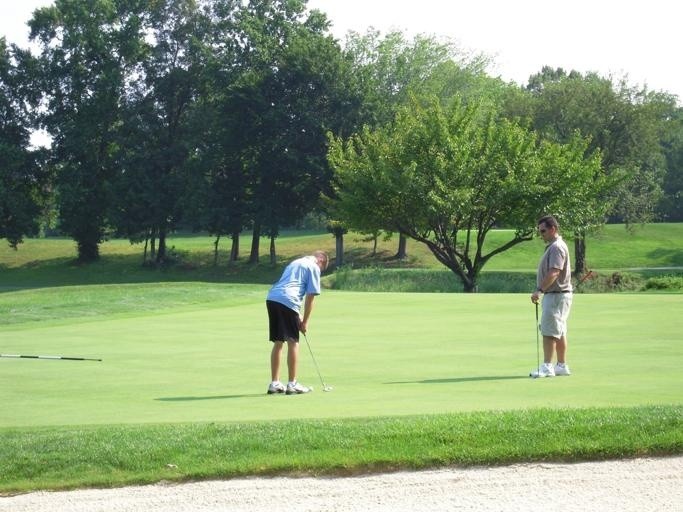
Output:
(543, 289), (573, 294)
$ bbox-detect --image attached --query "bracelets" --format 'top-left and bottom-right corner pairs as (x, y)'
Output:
(536, 286), (543, 293)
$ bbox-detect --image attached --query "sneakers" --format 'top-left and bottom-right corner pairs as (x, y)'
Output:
(529, 364), (555, 378)
(267, 382), (287, 394)
(284, 382), (310, 396)
(554, 365), (571, 377)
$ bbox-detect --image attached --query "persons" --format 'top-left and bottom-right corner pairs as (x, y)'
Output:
(265, 250), (330, 395)
(527, 215), (573, 377)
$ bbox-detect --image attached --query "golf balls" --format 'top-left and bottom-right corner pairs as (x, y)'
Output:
(328, 387), (331, 390)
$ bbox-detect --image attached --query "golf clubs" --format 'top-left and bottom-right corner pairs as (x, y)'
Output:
(303, 332), (329, 392)
(534, 303), (544, 378)
